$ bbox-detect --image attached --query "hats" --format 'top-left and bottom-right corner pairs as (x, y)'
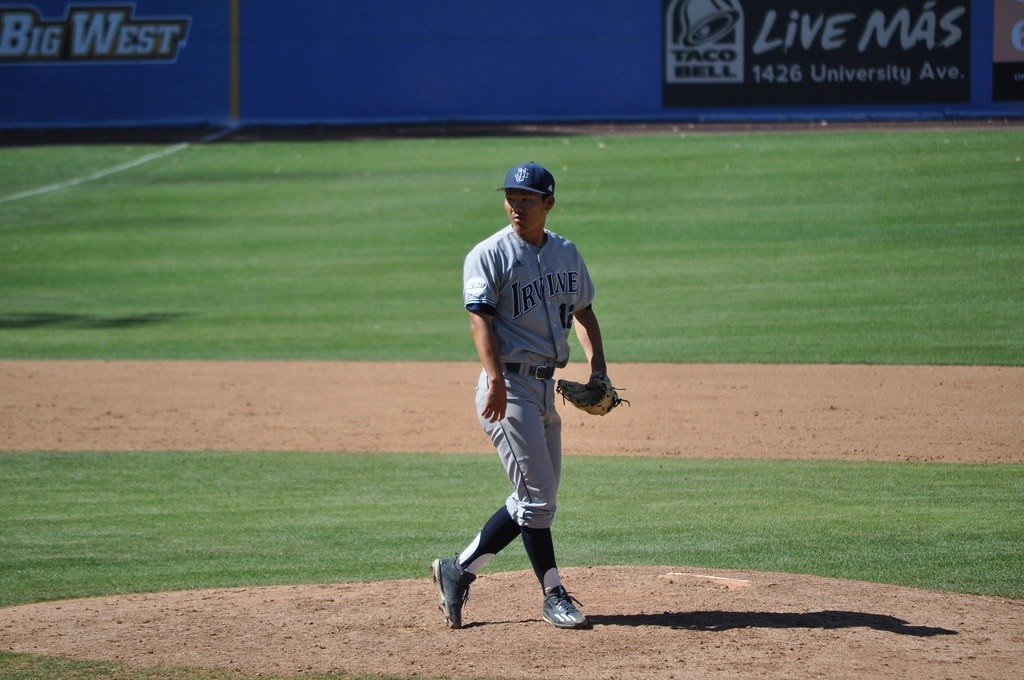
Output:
(497, 162), (555, 195)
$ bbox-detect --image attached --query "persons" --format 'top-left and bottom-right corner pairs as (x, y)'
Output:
(431, 162), (620, 629)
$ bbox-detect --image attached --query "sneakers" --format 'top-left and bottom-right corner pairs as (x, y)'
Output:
(430, 552), (476, 629)
(542, 585), (588, 627)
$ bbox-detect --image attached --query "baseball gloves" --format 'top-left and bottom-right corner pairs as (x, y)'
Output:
(555, 372), (621, 416)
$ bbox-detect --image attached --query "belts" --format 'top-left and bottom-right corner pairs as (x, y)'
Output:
(505, 363), (554, 380)
